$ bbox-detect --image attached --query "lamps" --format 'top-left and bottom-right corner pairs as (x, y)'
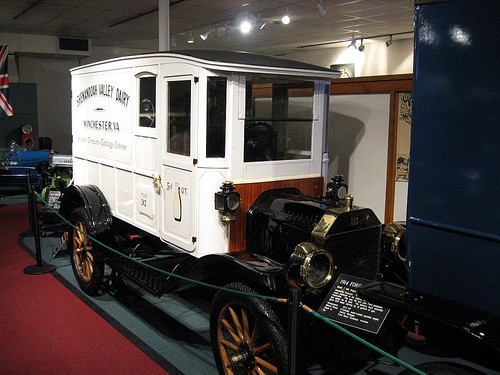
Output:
(282, 6), (292, 25)
(170, 34), (176, 48)
(349, 38), (365, 52)
(188, 29), (195, 44)
(386, 34), (392, 47)
(199, 21), (232, 42)
(316, 0), (329, 16)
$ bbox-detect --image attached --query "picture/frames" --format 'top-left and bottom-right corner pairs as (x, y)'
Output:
(330, 63), (354, 78)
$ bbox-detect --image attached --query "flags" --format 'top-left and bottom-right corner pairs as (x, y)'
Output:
(0, 43), (15, 119)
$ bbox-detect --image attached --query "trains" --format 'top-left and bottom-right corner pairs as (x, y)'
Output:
(56, 49), (407, 375)
